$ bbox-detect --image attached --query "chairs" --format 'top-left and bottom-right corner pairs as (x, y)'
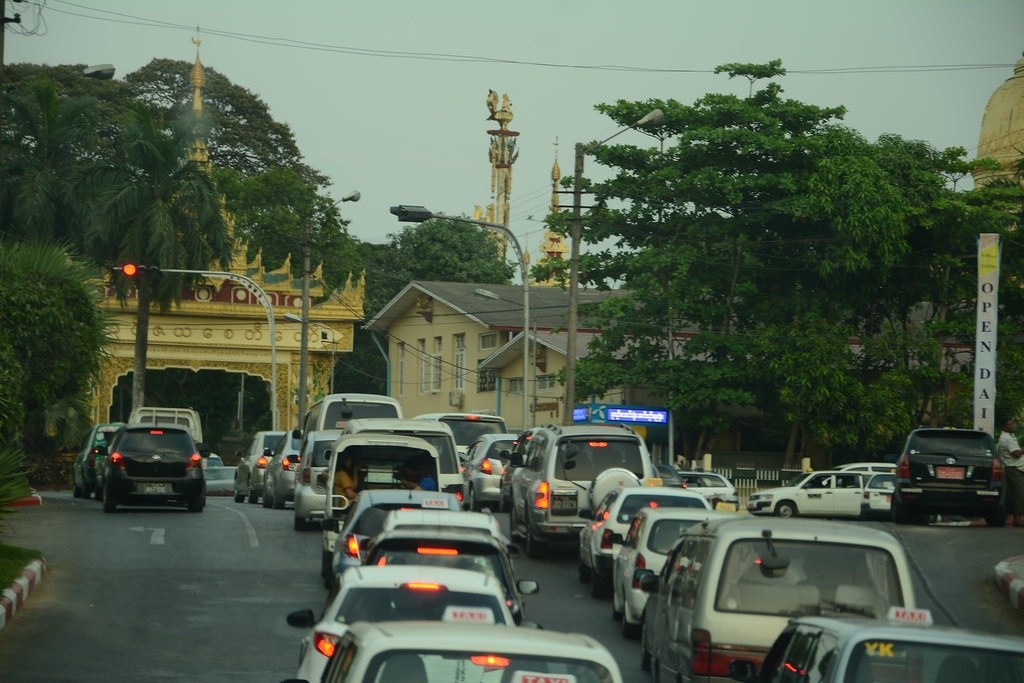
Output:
(937, 656), (982, 683)
(726, 565), (879, 614)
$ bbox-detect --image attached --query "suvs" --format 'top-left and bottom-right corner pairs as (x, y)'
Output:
(884, 424), (1007, 527)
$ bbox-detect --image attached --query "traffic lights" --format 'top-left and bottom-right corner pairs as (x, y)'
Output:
(123, 264), (160, 280)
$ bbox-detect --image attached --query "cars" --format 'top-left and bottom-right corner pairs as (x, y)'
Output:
(95, 422), (210, 511)
(860, 472), (899, 516)
(69, 407), (223, 499)
(728, 604), (1024, 683)
(744, 470), (878, 520)
(211, 390), (740, 683)
(819, 463), (899, 488)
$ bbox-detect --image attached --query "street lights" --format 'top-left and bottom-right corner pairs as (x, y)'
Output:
(564, 108), (666, 425)
(475, 287), (537, 428)
(296, 191), (362, 430)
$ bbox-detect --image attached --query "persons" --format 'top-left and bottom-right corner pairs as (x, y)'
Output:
(398, 461), (437, 491)
(333, 456), (359, 515)
(997, 416), (1024, 526)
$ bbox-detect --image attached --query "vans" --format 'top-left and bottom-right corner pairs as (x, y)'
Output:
(639, 516), (918, 683)
